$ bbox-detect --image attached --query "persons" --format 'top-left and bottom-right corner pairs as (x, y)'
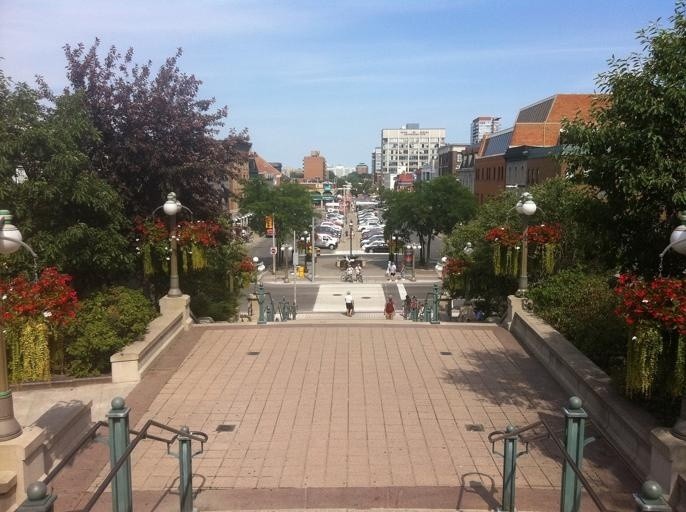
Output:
(399, 261), (407, 280)
(389, 261), (396, 281)
(383, 295), (395, 320)
(345, 264), (353, 281)
(410, 295), (417, 312)
(471, 305), (481, 321)
(354, 262), (362, 281)
(403, 295), (411, 318)
(344, 290), (354, 317)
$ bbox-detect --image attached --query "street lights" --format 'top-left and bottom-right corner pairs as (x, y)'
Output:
(665, 211), (686, 443)
(403, 240), (422, 282)
(347, 218), (354, 259)
(298, 230), (309, 274)
(248, 254), (266, 296)
(511, 189), (537, 299)
(231, 214), (253, 230)
(0, 208), (22, 444)
(159, 186), (187, 298)
(279, 242), (292, 283)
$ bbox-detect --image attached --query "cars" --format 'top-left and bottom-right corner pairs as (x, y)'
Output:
(306, 193), (392, 253)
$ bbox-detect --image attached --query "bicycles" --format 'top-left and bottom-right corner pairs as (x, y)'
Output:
(355, 273), (363, 283)
(338, 272), (352, 282)
